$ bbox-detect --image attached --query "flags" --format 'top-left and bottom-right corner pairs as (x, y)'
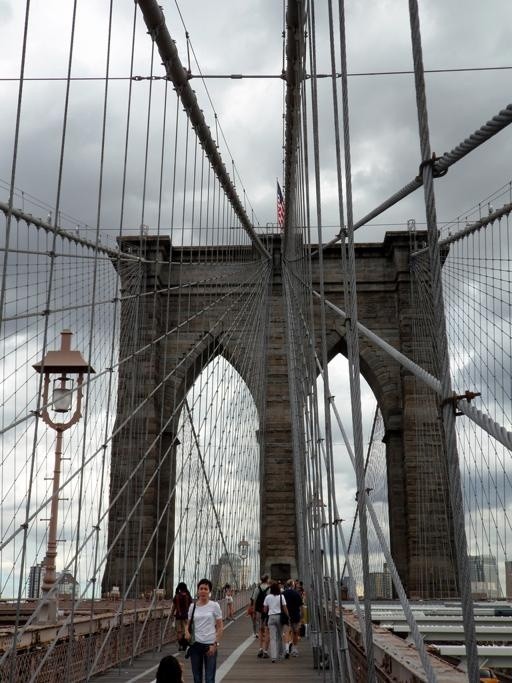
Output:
(277, 181), (285, 229)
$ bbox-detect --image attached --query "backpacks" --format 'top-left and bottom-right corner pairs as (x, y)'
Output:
(255, 585), (270, 614)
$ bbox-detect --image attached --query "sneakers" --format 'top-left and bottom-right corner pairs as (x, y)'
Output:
(257, 640), (299, 663)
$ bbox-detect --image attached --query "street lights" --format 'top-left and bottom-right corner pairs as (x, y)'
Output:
(31, 328), (97, 626)
(238, 535), (249, 590)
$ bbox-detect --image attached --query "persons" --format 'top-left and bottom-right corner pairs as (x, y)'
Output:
(171, 578), (223, 683)
(225, 585), (236, 620)
(155, 655), (183, 683)
(248, 575), (304, 663)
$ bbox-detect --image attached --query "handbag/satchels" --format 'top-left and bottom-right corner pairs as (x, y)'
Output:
(299, 624), (306, 637)
(188, 602), (196, 645)
(280, 593), (289, 625)
